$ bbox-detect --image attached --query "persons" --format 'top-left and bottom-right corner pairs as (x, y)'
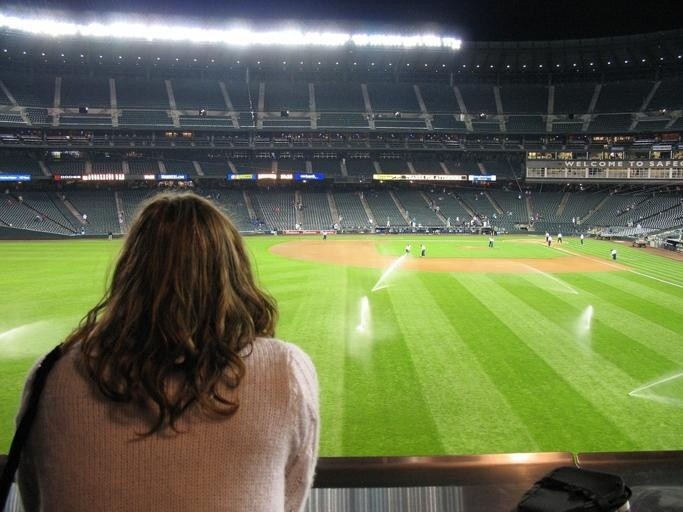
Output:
(17, 188), (320, 511)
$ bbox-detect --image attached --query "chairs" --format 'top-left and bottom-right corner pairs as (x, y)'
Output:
(0, 58), (682, 252)
